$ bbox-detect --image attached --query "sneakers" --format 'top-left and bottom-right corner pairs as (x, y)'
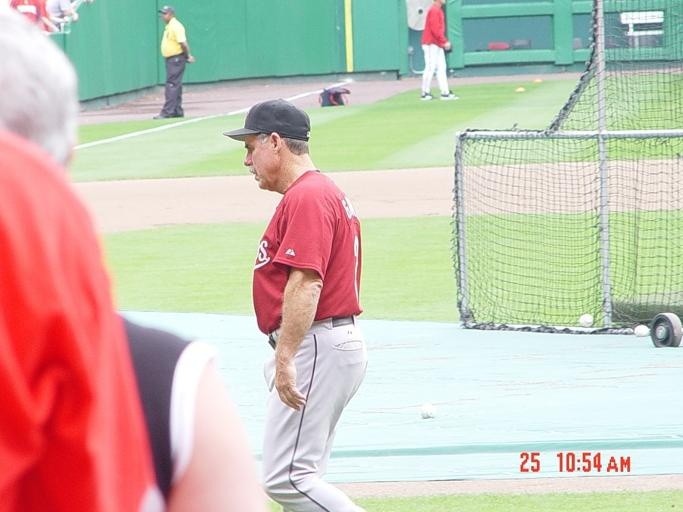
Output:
(151, 112), (184, 121)
(418, 90), (460, 102)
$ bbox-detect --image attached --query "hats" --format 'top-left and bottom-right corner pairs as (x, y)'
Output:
(156, 5), (175, 15)
(222, 98), (315, 141)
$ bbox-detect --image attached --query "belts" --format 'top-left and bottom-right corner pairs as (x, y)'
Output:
(265, 312), (355, 352)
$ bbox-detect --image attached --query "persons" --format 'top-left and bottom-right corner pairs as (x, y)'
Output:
(0, 127), (169, 512)
(420, 0), (461, 101)
(151, 4), (195, 119)
(8, 0), (83, 36)
(0, 0), (285, 512)
(222, 99), (371, 512)
